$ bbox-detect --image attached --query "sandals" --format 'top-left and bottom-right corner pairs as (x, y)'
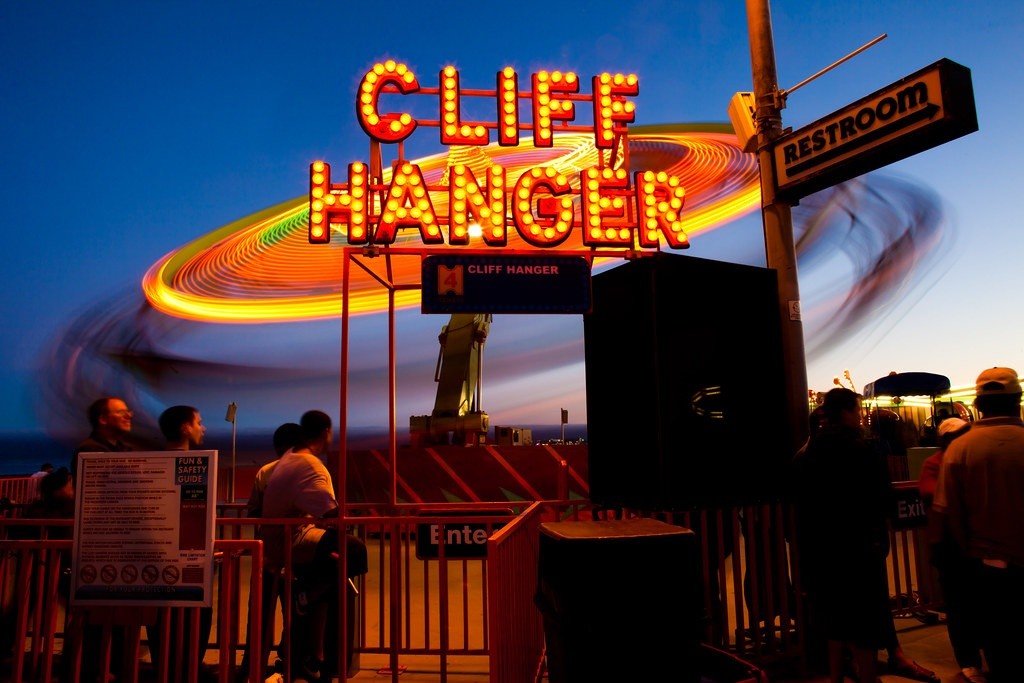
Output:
(887, 659), (941, 683)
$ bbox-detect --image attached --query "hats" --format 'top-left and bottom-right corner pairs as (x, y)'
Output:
(976, 367), (1022, 396)
(938, 417), (968, 437)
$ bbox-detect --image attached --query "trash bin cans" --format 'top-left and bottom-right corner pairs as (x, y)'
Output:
(536, 517), (706, 683)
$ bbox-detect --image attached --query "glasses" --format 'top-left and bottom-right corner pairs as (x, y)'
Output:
(105, 409), (133, 417)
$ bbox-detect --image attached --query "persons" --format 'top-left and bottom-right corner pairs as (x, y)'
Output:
(27, 462), (53, 502)
(144, 404), (212, 683)
(235, 421), (305, 682)
(936, 366), (1023, 683)
(796, 388), (892, 683)
(70, 395), (145, 683)
(790, 405), (951, 683)
(911, 416), (972, 624)
(0, 467), (79, 681)
(258, 409), (355, 683)
